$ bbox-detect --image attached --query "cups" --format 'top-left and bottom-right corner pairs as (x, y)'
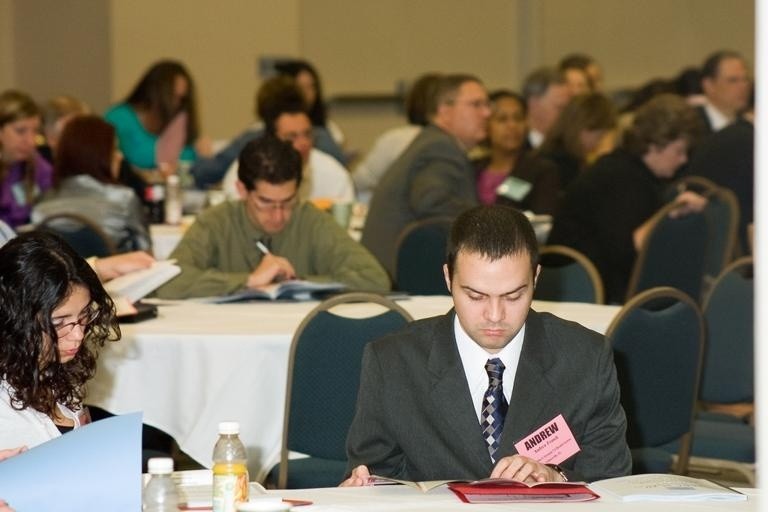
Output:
(207, 191), (227, 208)
(330, 202), (351, 230)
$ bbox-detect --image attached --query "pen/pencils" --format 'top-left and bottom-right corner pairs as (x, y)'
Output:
(254, 239), (273, 260)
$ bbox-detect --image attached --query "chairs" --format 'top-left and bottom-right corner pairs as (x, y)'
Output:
(667, 257), (754, 463)
(620, 179), (738, 312)
(608, 286), (701, 474)
(393, 223), (457, 295)
(32, 212), (112, 258)
(530, 246), (604, 307)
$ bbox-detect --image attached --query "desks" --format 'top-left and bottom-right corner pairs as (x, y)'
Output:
(148, 213), (553, 262)
(82, 296), (622, 487)
(182, 486), (755, 512)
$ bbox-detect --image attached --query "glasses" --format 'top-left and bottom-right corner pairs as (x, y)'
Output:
(251, 196), (297, 213)
(40, 302), (102, 339)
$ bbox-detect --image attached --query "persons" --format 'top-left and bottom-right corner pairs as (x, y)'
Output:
(0, 51), (753, 306)
(0, 446), (27, 512)
(0, 230), (121, 450)
(337, 203), (633, 487)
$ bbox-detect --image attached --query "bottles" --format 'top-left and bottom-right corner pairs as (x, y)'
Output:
(163, 174), (182, 225)
(142, 458), (181, 512)
(209, 420), (248, 511)
(145, 165), (165, 221)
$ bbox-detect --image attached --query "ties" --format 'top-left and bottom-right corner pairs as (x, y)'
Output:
(476, 356), (509, 460)
